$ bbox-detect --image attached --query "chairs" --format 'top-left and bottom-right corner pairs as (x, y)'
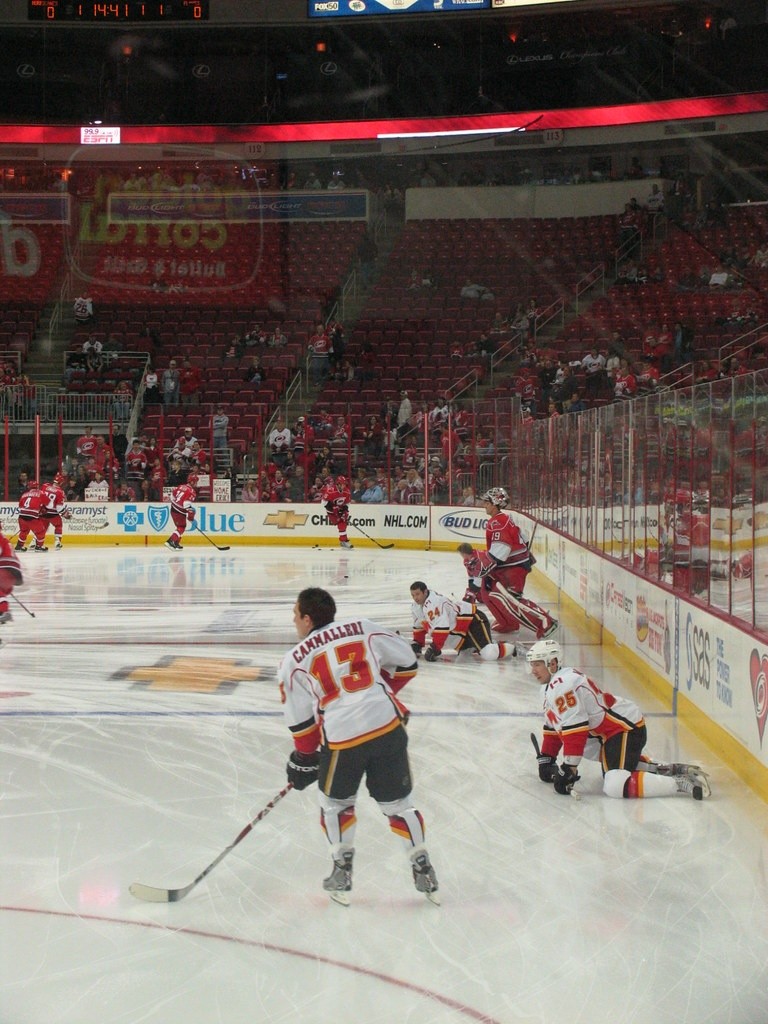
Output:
(0, 157), (768, 509)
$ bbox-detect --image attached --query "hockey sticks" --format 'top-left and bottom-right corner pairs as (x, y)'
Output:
(395, 630), (453, 663)
(9, 593), (35, 618)
(187, 517), (230, 550)
(530, 732), (581, 801)
(9, 530), (20, 541)
(529, 519), (539, 551)
(72, 517), (109, 529)
(347, 520), (395, 550)
(129, 782), (295, 904)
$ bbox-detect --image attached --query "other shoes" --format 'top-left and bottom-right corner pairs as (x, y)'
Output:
(28, 545), (36, 549)
(538, 623), (560, 641)
(511, 641), (530, 660)
(54, 541), (63, 550)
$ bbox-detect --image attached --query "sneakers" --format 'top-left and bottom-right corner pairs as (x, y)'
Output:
(339, 541), (355, 551)
(322, 847), (355, 906)
(14, 544), (28, 552)
(35, 546), (48, 552)
(409, 849), (442, 906)
(165, 537), (183, 551)
(656, 763), (711, 800)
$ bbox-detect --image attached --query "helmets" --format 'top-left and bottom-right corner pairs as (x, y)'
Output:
(28, 480), (38, 488)
(187, 475), (199, 483)
(526, 640), (563, 676)
(53, 476), (65, 485)
(480, 487), (510, 509)
(336, 476), (346, 484)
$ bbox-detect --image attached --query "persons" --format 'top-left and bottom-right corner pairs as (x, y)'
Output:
(526, 640), (712, 800)
(619, 489), (753, 596)
(0, 156), (768, 553)
(0, 521), (24, 624)
(410, 581), (518, 662)
(277, 588), (441, 907)
(466, 487), (559, 640)
(457, 543), (490, 604)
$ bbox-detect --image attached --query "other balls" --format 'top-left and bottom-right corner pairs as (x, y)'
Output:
(330, 549), (334, 551)
(116, 543), (119, 545)
(344, 576), (348, 578)
(312, 544), (321, 550)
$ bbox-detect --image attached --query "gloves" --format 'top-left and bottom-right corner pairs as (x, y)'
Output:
(425, 643), (442, 662)
(554, 762), (581, 794)
(410, 640), (423, 658)
(325, 501), (334, 512)
(536, 755), (564, 783)
(285, 751), (321, 790)
